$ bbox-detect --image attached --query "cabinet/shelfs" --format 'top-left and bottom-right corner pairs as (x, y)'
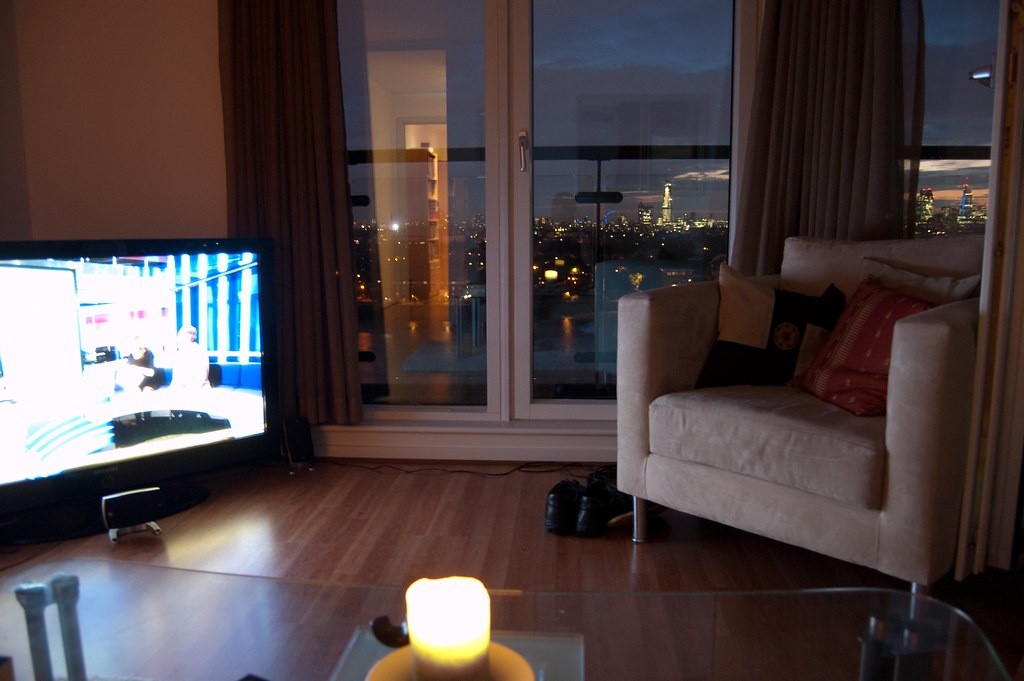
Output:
(406, 147), (444, 298)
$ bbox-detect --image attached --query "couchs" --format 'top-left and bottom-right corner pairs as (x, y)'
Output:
(611, 232), (984, 592)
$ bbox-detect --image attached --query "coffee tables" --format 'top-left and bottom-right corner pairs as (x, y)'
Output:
(0, 555), (1012, 681)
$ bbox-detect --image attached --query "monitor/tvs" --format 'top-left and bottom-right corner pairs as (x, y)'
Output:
(1, 236), (284, 516)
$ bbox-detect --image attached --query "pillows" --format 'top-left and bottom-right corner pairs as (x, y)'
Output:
(697, 262), (845, 392)
(789, 273), (938, 418)
(857, 257), (981, 308)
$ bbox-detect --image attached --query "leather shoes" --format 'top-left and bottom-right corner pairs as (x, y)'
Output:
(545, 479), (585, 530)
(578, 478), (668, 538)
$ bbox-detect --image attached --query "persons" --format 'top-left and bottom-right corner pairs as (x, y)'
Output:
(163, 323), (213, 390)
(110, 332), (162, 396)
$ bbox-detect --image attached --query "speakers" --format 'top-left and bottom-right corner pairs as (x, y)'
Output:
(282, 416), (315, 461)
